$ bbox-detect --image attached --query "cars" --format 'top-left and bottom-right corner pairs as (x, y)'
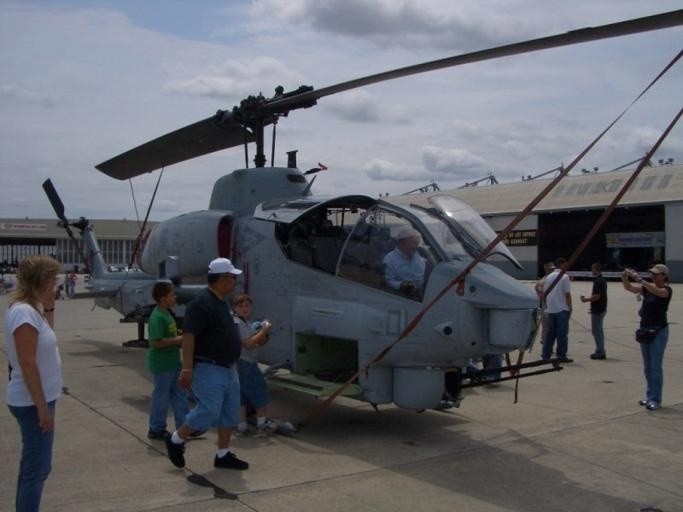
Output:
(106, 265), (141, 272)
(0, 264), (18, 274)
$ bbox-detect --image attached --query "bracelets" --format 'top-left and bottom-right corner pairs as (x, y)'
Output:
(43, 308), (54, 312)
(635, 277), (641, 282)
(180, 367), (193, 373)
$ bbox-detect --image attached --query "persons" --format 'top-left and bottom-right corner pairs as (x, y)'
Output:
(539, 259), (573, 363)
(535, 261), (556, 356)
(382, 228), (427, 297)
(620, 261), (673, 409)
(69, 271), (77, 295)
(63, 270), (69, 296)
(232, 294), (278, 434)
(147, 281), (207, 438)
(165, 258), (250, 469)
(2, 256), (61, 511)
(579, 263), (608, 358)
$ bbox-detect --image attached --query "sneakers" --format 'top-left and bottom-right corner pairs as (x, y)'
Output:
(215, 451), (248, 469)
(164, 432), (185, 467)
(236, 423), (257, 436)
(149, 428), (169, 439)
(256, 417), (282, 433)
(640, 400), (661, 409)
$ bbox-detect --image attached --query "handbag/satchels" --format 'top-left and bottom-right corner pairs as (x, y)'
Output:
(636, 329), (658, 342)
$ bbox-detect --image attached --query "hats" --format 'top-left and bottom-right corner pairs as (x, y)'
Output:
(649, 264), (668, 273)
(208, 258), (242, 275)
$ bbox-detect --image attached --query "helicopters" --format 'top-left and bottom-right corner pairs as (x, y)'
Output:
(41, 9), (682, 441)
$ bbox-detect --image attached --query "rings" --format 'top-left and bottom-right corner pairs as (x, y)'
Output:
(177, 376), (182, 380)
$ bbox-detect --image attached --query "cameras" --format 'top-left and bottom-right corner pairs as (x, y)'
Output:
(625, 262), (632, 270)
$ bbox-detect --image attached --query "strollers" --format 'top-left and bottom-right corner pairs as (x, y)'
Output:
(53, 282), (65, 300)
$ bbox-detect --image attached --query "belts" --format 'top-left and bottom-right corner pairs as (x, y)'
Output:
(194, 358), (221, 364)
